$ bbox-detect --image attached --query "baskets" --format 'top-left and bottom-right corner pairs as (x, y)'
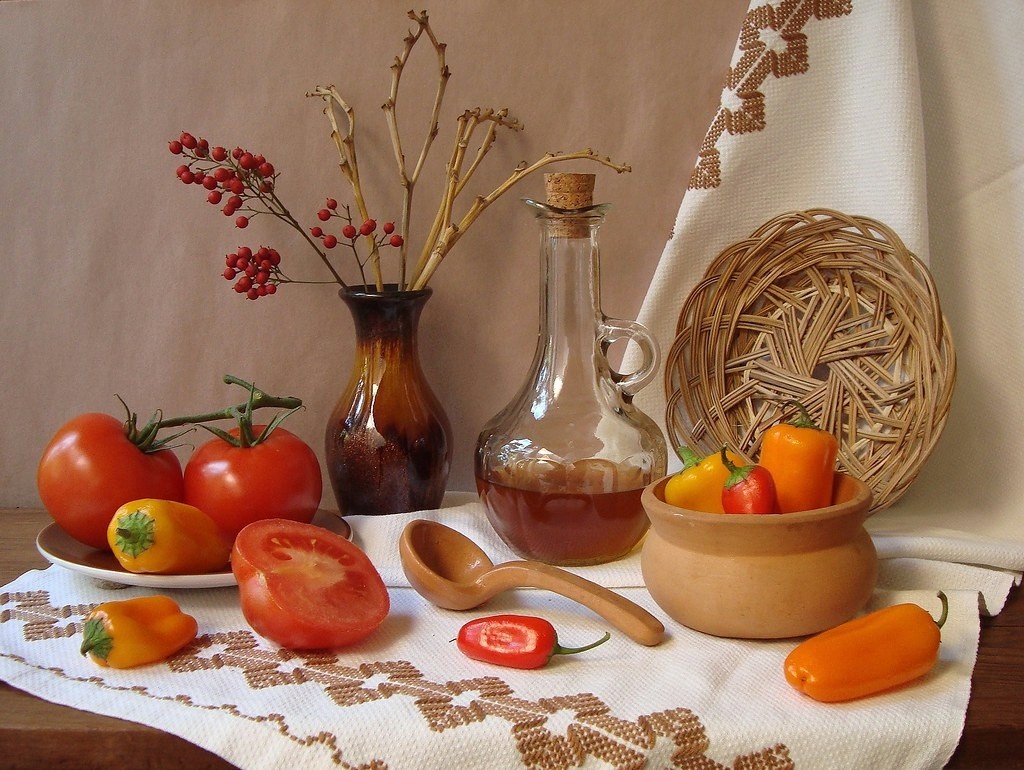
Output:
(666, 209), (955, 516)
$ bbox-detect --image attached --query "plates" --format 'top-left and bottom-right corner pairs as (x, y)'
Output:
(37, 508), (354, 587)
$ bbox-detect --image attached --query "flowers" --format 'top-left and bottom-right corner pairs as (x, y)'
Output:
(163, 4), (640, 304)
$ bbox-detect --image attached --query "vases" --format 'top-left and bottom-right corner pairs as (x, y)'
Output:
(326, 283), (451, 522)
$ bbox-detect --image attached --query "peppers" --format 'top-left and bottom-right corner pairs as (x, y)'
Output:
(650, 401), (838, 515)
(784, 586), (949, 697)
(76, 597), (203, 673)
(455, 616), (610, 669)
(106, 497), (227, 574)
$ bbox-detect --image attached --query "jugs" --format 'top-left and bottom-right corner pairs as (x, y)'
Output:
(473, 196), (667, 566)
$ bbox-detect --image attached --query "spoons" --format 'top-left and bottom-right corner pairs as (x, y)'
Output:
(399, 520), (664, 646)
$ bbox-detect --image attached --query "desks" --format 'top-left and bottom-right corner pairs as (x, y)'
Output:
(0, 501), (1022, 769)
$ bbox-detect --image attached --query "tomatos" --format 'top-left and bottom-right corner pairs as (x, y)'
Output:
(188, 376), (326, 543)
(34, 380), (186, 552)
(231, 514), (390, 652)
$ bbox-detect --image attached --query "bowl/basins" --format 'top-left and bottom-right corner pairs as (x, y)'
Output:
(639, 472), (878, 639)
